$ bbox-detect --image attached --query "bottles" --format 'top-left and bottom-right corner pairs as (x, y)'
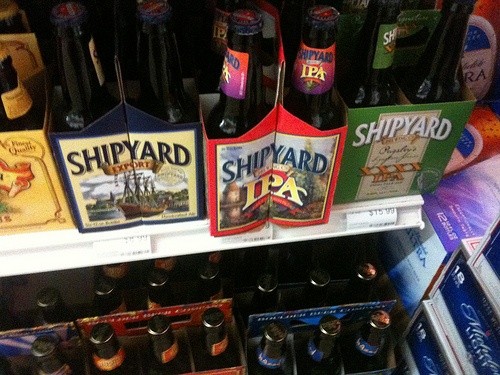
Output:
(191, 259), (224, 302)
(392, 0), (478, 103)
(0, 1), (32, 34)
(31, 333), (76, 375)
(92, 277), (128, 316)
(48, 2), (118, 132)
(297, 315), (342, 375)
(247, 321), (289, 375)
(345, 308), (392, 373)
(32, 287), (69, 327)
(128, 0), (198, 124)
(205, 7), (268, 139)
(195, 308), (236, 371)
(342, 263), (377, 304)
(300, 267), (335, 308)
(240, 273), (281, 334)
(146, 314), (187, 375)
(145, 268), (170, 310)
(333, 0), (401, 109)
(284, 5), (339, 130)
(0, 47), (41, 132)
(89, 322), (133, 375)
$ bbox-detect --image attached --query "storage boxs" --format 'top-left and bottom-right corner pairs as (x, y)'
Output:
(0, 0), (500, 375)
